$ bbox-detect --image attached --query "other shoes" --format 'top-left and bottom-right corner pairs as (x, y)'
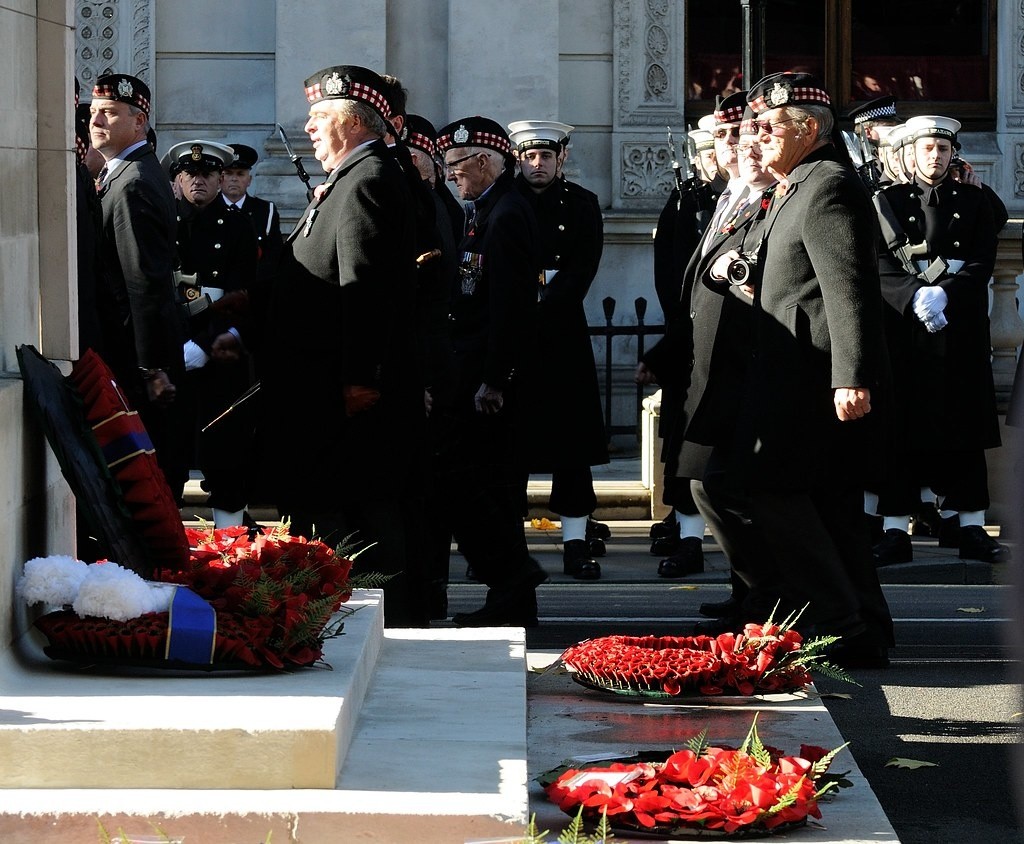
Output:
(452, 600), (539, 627)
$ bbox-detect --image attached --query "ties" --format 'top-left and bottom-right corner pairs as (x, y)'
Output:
(95, 163), (109, 194)
(708, 188), (731, 240)
(230, 204), (238, 217)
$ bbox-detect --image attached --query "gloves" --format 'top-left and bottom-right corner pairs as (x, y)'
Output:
(913, 285), (949, 335)
(200, 286), (223, 303)
(182, 339), (210, 371)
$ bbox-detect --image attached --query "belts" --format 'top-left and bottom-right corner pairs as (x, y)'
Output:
(918, 258), (964, 275)
(539, 269), (559, 285)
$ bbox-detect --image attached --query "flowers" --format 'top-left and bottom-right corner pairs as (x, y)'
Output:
(546, 709), (854, 833)
(562, 623), (863, 699)
(15, 343), (404, 674)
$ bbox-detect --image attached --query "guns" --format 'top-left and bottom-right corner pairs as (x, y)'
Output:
(860, 123), (947, 289)
(666, 125), (682, 189)
(274, 123), (316, 195)
(683, 125), (699, 196)
(840, 128), (882, 194)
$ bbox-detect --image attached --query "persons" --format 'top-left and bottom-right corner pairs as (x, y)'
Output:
(642, 69), (894, 668)
(436, 115), (616, 632)
(847, 96), (1005, 566)
(75, 72), (282, 538)
(275, 63), (464, 630)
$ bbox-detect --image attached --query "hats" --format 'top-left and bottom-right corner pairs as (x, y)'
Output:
(400, 114), (576, 173)
(73, 72), (158, 163)
(875, 114), (968, 168)
(846, 94), (903, 123)
(304, 65), (397, 120)
(161, 139), (258, 183)
(688, 70), (832, 153)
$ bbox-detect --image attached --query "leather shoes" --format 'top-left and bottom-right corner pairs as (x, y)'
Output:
(869, 509), (1013, 563)
(649, 508), (705, 576)
(563, 521), (610, 580)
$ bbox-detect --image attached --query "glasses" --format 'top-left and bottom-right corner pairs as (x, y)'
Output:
(445, 152), (492, 174)
(712, 128), (740, 139)
(731, 143), (762, 156)
(750, 118), (794, 135)
